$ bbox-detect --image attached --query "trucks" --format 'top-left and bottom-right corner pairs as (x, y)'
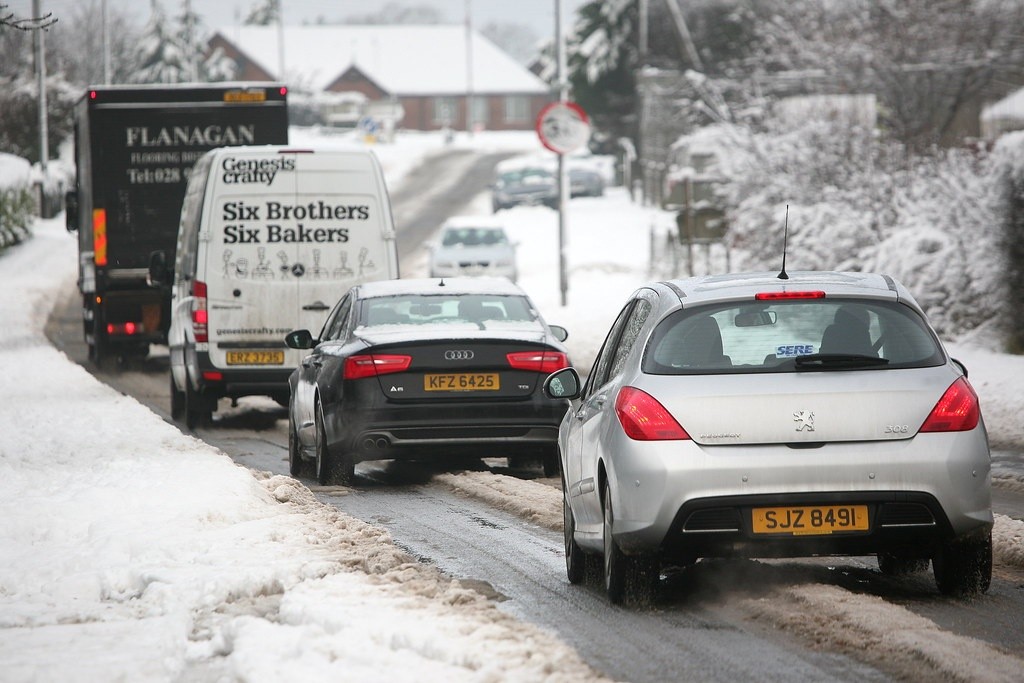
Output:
(167, 145), (400, 439)
(64, 83), (288, 368)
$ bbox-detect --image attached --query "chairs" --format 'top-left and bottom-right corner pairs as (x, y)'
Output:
(821, 324), (878, 358)
(673, 318), (732, 367)
(466, 306), (505, 321)
(366, 308), (411, 322)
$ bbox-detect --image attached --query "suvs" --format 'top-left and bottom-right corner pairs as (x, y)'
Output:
(541, 271), (994, 609)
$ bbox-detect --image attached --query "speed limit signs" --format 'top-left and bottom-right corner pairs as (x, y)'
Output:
(537, 101), (589, 155)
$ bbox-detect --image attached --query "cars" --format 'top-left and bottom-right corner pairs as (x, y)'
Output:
(424, 222), (518, 283)
(567, 163), (603, 197)
(282, 274), (572, 484)
(489, 156), (561, 214)
